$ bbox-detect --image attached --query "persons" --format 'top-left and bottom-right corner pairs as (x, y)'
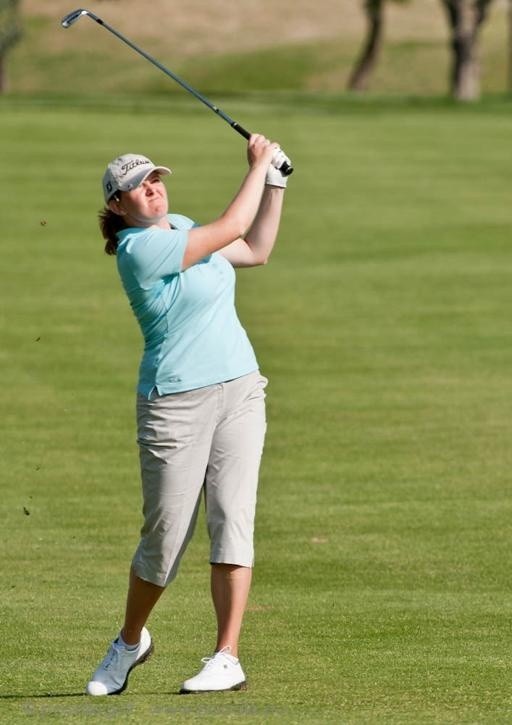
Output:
(85, 134), (293, 698)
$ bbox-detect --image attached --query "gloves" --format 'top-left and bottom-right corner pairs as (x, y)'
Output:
(265, 142), (291, 188)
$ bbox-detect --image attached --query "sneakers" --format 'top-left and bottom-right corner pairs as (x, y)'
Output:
(86, 627), (154, 698)
(178, 645), (248, 692)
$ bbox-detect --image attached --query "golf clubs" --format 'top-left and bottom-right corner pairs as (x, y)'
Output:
(62, 9), (292, 175)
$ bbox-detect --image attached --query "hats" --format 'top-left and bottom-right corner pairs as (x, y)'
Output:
(102, 152), (172, 204)
